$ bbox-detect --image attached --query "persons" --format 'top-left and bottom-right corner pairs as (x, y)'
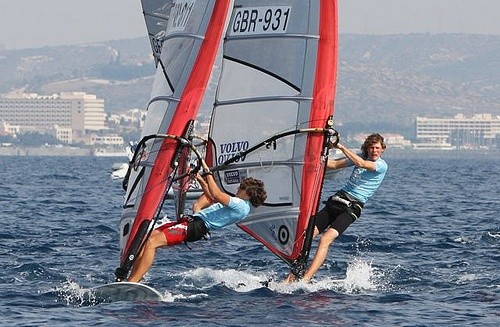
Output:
(128, 158), (268, 283)
(280, 132), (388, 283)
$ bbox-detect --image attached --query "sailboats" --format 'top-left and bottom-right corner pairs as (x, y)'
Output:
(141, 1), (339, 281)
(76, 2), (235, 307)
